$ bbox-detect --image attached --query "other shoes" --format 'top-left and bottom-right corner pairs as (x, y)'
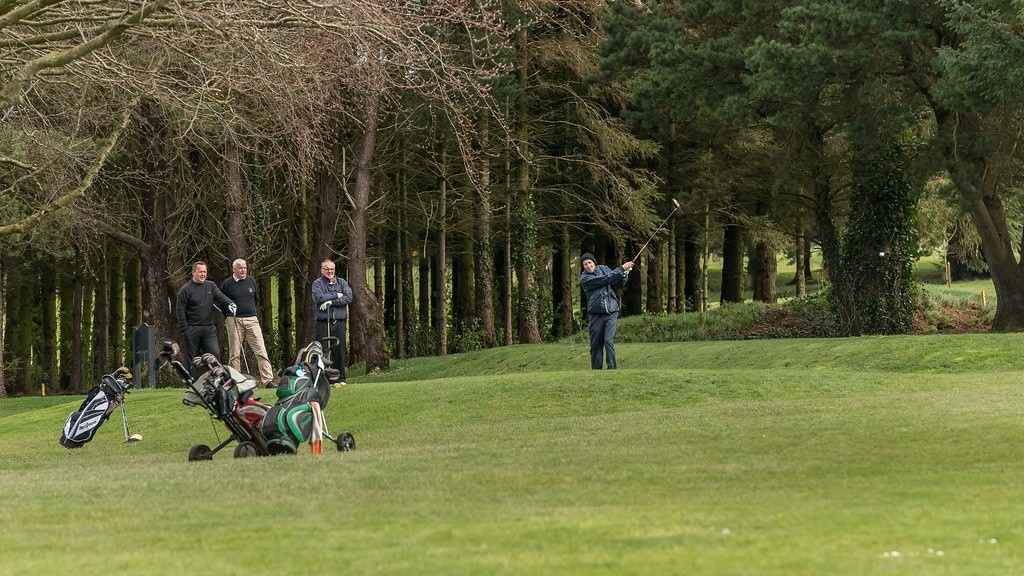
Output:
(266, 381), (278, 388)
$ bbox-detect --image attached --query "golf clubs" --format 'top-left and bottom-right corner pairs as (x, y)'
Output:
(632, 198), (681, 263)
(232, 310), (251, 375)
(326, 303), (333, 369)
(153, 336), (232, 408)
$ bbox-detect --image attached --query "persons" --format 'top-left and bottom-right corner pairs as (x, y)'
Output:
(311, 259), (353, 386)
(579, 252), (634, 369)
(176, 261), (237, 381)
(219, 259), (278, 388)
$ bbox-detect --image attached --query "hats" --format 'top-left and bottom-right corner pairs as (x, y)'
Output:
(580, 253), (597, 265)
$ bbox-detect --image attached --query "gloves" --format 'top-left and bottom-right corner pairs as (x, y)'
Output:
(320, 301), (332, 310)
(337, 293), (343, 298)
(229, 302), (237, 316)
